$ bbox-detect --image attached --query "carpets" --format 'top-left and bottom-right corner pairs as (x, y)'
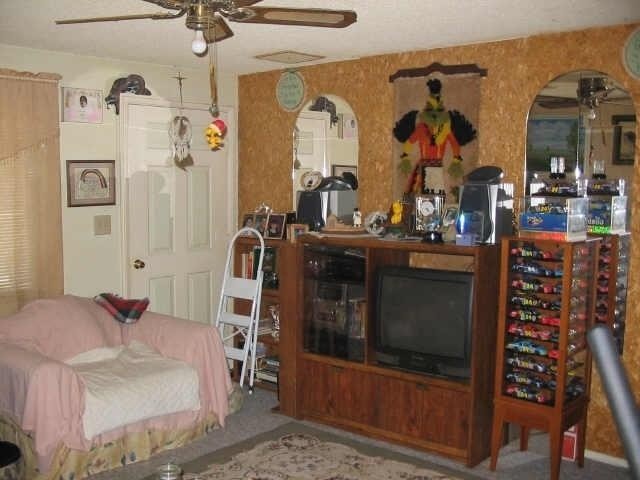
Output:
(142, 420), (488, 480)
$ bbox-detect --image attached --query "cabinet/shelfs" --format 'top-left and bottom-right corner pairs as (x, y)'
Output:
(294, 235), (500, 469)
(233, 239), (281, 393)
(490, 236), (601, 480)
(586, 228), (631, 354)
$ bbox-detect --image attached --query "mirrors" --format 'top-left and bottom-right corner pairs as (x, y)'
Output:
(293, 94), (358, 231)
(525, 68), (636, 356)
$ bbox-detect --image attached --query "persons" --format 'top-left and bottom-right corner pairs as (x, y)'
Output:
(257, 217), (267, 235)
(79, 96), (89, 114)
(244, 216), (252, 235)
(268, 220), (280, 238)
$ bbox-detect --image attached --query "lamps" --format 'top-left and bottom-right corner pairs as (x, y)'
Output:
(191, 23), (207, 54)
(577, 98), (601, 119)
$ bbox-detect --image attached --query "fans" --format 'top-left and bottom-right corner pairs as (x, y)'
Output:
(53, 0), (358, 45)
(535, 81), (633, 108)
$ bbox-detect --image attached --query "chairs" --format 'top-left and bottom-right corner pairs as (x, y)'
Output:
(0, 295), (242, 480)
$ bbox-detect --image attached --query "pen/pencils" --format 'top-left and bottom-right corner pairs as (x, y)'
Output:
(402, 192), (412, 202)
(425, 188), (445, 194)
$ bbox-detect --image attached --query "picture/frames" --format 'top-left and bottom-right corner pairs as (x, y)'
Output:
(66, 160), (116, 207)
(333, 165), (357, 181)
(526, 113), (591, 177)
(612, 115), (635, 166)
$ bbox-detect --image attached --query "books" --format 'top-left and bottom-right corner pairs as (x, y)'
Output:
(242, 318), (274, 336)
(241, 244), (276, 288)
(237, 339), (280, 384)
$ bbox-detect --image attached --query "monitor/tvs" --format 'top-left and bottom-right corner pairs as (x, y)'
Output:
(375, 265), (474, 383)
